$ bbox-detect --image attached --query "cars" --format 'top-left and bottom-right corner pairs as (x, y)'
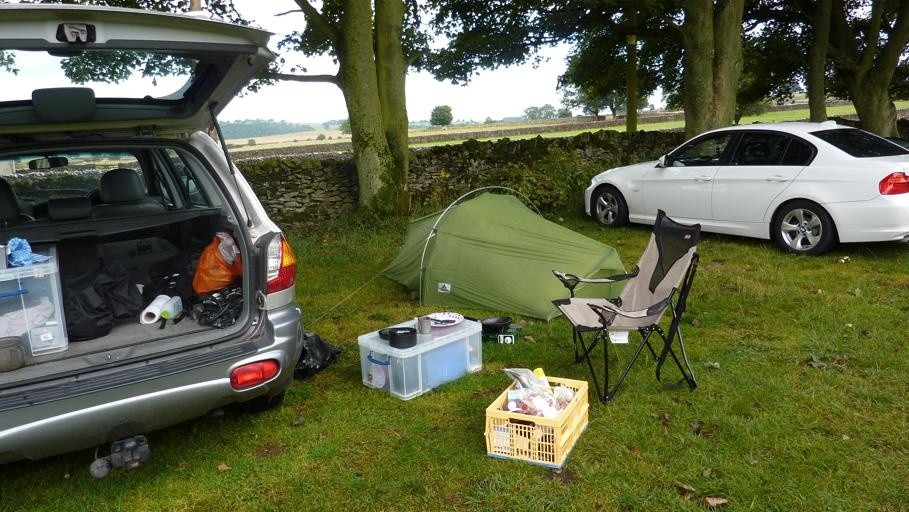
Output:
(585, 121), (909, 253)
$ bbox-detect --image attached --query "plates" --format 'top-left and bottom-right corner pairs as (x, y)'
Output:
(426, 312), (465, 328)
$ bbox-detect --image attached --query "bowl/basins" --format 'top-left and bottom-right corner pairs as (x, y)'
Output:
(482, 317), (513, 333)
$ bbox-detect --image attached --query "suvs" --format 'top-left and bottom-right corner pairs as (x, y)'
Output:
(0, 4), (302, 465)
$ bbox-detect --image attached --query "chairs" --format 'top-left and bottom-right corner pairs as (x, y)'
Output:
(48, 197), (92, 220)
(94, 169), (168, 217)
(551, 209), (701, 403)
(0, 178), (23, 226)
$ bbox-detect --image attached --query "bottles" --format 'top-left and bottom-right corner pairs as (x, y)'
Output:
(159, 295), (183, 320)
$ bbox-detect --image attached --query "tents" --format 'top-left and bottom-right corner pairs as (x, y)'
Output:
(381, 189), (635, 327)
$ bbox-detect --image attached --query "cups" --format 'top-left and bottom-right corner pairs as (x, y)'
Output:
(0, 336), (27, 372)
(416, 316), (432, 334)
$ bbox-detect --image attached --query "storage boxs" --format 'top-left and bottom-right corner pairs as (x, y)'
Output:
(357, 319), (483, 401)
(0, 245), (71, 357)
